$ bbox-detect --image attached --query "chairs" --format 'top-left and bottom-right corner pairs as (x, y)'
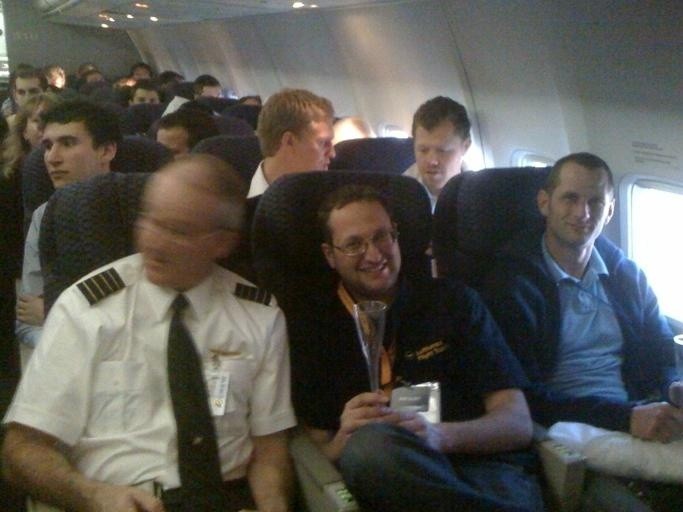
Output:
(0, 75), (588, 512)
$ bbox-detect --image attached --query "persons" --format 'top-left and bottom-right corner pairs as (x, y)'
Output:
(218, 89), (335, 288)
(14, 96), (120, 349)
(0, 59), (263, 160)
(1, 153), (295, 512)
(480, 149), (683, 511)
(400, 93), (471, 215)
(282, 182), (542, 510)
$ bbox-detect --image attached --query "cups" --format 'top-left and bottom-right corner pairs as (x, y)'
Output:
(673, 334), (683, 383)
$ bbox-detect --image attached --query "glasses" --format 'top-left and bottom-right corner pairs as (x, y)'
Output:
(330, 221), (398, 257)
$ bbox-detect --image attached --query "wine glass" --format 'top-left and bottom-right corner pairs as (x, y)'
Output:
(353, 300), (389, 391)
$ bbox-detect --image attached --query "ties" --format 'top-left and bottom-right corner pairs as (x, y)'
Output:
(168, 294), (226, 511)
